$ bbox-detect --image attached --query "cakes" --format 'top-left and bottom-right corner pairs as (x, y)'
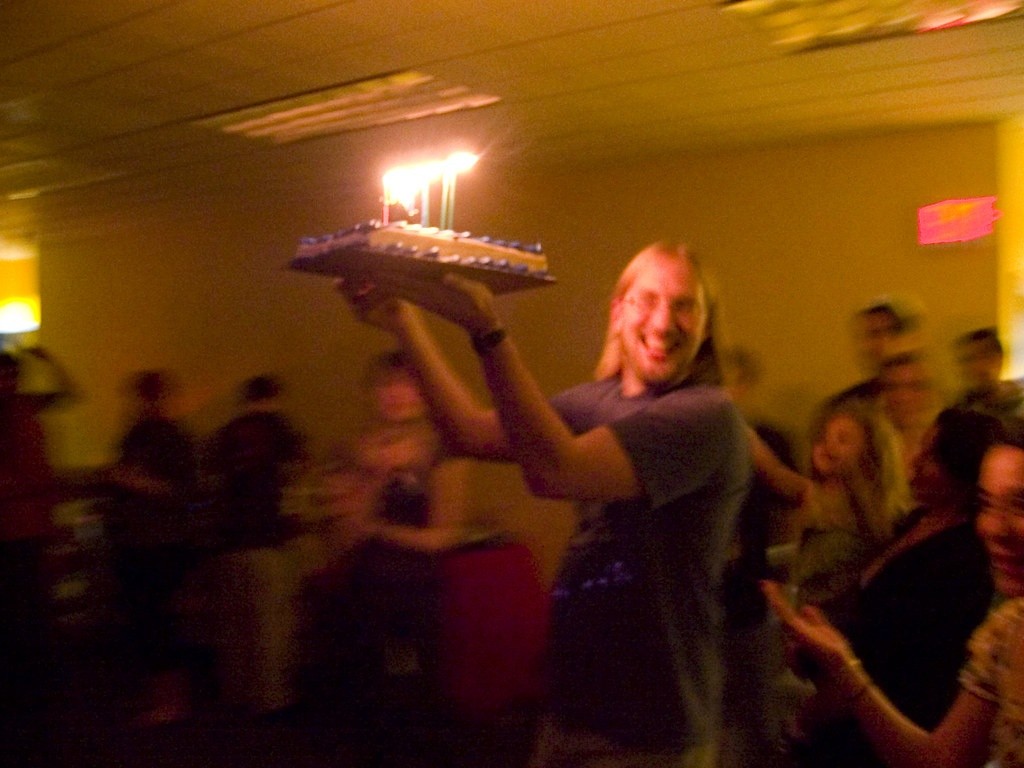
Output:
(290, 219), (549, 278)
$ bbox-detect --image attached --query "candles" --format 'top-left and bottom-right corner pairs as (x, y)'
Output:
(382, 168), (459, 230)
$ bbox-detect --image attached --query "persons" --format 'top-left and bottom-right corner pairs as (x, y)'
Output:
(0, 336), (466, 768)
(334, 242), (750, 768)
(725, 298), (1024, 768)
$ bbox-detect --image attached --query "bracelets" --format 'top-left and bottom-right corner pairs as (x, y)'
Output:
(833, 658), (860, 682)
(848, 680), (878, 699)
(471, 327), (507, 354)
